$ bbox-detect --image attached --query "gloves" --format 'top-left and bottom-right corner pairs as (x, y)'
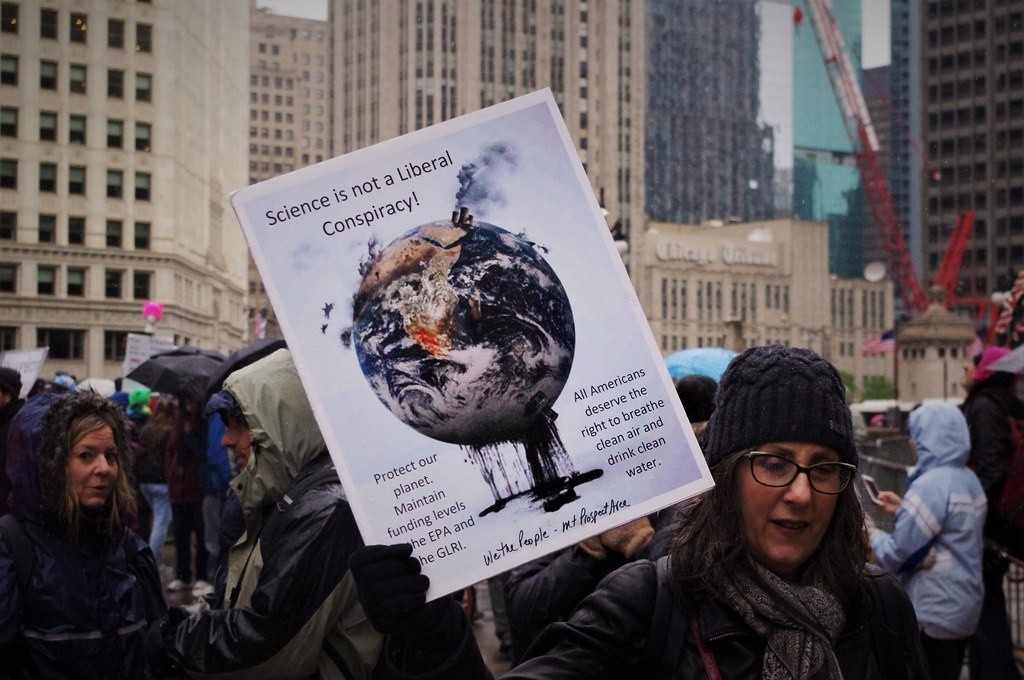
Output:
(346, 540), (428, 632)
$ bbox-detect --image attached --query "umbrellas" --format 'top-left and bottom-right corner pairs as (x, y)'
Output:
(152, 346), (227, 362)
(198, 337), (287, 403)
(664, 345), (739, 382)
(125, 353), (221, 401)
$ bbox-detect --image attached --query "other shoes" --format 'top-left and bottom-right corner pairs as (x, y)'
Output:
(167, 579), (191, 592)
(190, 581), (214, 599)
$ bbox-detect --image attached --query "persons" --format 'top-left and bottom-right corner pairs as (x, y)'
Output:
(0, 297), (1024, 680)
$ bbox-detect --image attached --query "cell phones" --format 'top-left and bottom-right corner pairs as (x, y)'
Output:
(861, 474), (883, 506)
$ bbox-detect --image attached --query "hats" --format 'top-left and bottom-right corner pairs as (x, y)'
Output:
(677, 373), (722, 423)
(699, 344), (887, 472)
(0, 367), (21, 396)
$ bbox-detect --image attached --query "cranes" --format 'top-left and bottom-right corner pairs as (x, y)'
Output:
(931, 209), (978, 309)
(790, 0), (929, 318)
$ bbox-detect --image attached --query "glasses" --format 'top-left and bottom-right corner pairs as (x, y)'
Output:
(742, 447), (859, 495)
